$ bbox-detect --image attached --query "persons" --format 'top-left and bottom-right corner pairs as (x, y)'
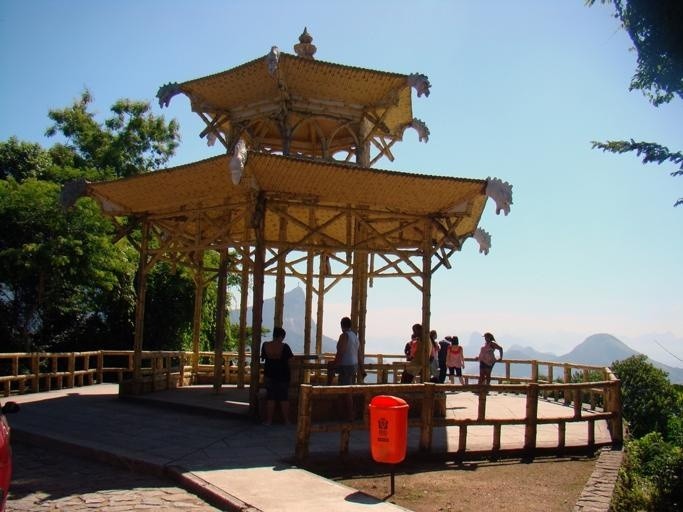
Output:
(260, 327), (294, 426)
(474, 333), (503, 384)
(399, 325), (465, 392)
(328, 318), (367, 421)
(0, 400), (13, 511)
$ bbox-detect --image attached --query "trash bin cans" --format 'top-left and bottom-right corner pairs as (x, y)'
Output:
(368, 395), (410, 464)
